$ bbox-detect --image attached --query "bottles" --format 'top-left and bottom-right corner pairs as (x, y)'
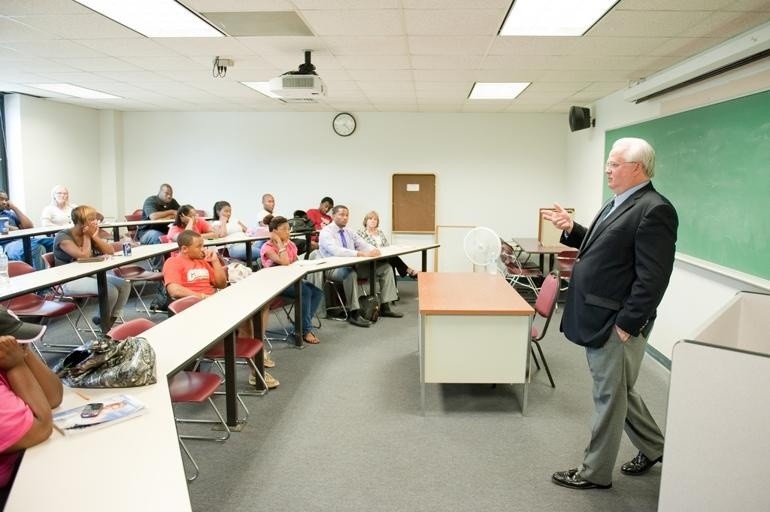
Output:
(0, 246), (10, 291)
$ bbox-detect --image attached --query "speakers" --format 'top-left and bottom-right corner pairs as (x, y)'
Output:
(568, 105), (591, 132)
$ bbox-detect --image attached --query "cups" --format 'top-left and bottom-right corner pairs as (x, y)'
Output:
(0, 217), (9, 235)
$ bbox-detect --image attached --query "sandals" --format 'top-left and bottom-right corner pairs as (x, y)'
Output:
(306, 333), (320, 343)
(409, 270), (417, 280)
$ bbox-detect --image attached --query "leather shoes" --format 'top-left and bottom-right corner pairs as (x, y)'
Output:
(380, 310), (403, 318)
(552, 468), (612, 489)
(621, 451), (662, 476)
(350, 316), (372, 327)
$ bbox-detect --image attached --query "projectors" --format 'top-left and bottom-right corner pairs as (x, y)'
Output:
(269, 74), (328, 104)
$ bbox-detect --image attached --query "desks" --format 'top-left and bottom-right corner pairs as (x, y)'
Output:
(0, 376), (199, 512)
(514, 240), (568, 269)
(298, 235), (441, 320)
(418, 271), (535, 415)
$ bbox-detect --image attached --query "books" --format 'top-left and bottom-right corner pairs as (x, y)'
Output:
(51, 394), (147, 436)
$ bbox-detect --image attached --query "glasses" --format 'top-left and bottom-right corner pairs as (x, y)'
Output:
(606, 161), (637, 169)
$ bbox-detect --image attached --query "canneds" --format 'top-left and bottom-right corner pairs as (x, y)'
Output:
(123, 242), (132, 257)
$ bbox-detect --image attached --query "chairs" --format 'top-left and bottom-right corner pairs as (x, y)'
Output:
(529, 270), (561, 388)
(501, 245), (542, 295)
(558, 249), (579, 284)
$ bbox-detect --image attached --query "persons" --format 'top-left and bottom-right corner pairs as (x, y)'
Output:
(541, 137), (678, 490)
(0, 304), (63, 510)
(319, 205), (403, 327)
(1, 184), (333, 344)
(355, 210), (418, 305)
(162, 229), (280, 389)
(53, 402), (127, 429)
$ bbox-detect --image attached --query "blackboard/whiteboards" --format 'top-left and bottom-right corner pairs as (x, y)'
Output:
(602, 89), (769, 290)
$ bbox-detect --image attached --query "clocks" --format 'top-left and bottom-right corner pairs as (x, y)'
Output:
(333, 113), (357, 135)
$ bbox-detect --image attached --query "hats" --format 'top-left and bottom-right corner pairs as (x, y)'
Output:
(294, 211), (307, 218)
(0, 305), (47, 343)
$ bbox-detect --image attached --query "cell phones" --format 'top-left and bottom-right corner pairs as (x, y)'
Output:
(80, 403), (104, 418)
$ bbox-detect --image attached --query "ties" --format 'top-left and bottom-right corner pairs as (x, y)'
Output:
(584, 200), (614, 246)
(339, 230), (348, 248)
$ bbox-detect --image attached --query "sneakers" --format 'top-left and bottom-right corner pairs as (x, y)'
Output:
(263, 352), (275, 367)
(249, 372), (280, 388)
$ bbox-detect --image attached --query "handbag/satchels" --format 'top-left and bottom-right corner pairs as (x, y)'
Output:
(288, 218), (316, 231)
(57, 339), (158, 388)
(359, 295), (379, 322)
(228, 262), (252, 285)
(150, 285), (170, 310)
